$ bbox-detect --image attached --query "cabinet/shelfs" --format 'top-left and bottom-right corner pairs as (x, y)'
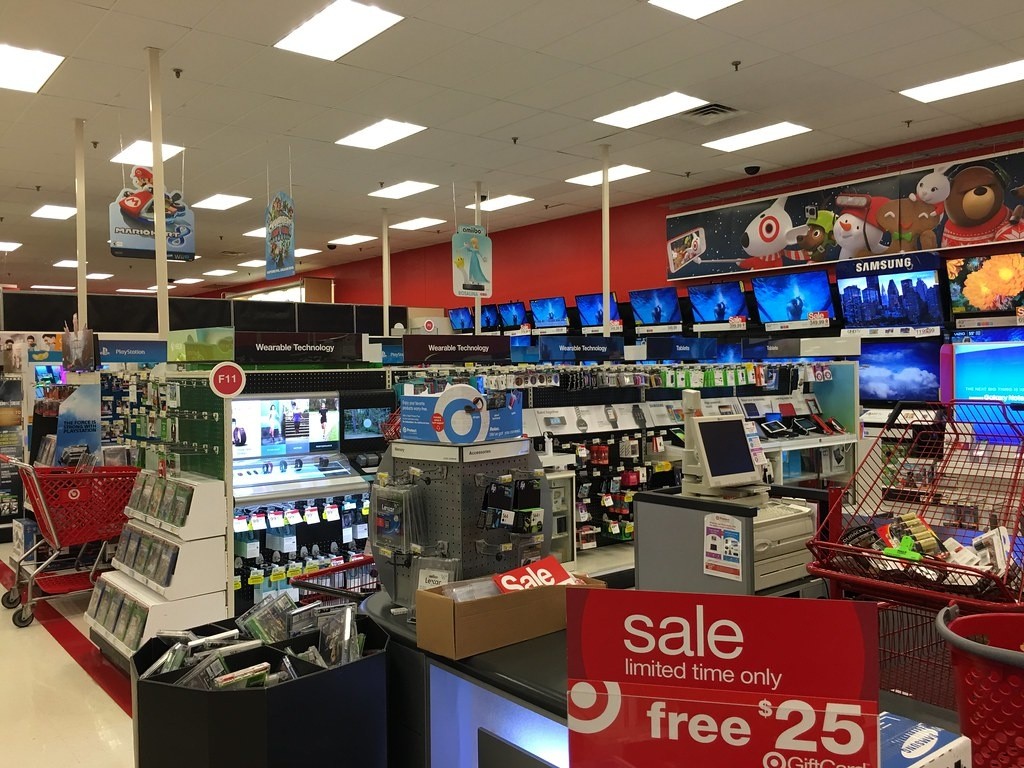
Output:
(0, 360), (1024, 681)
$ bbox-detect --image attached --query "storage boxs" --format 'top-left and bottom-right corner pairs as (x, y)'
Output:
(416, 574), (607, 660)
(400, 391), (523, 444)
(12, 518), (36, 562)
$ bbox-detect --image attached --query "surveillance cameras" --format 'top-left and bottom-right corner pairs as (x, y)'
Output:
(481, 195), (486, 202)
(327, 245), (336, 250)
(168, 279), (175, 283)
(745, 166), (761, 175)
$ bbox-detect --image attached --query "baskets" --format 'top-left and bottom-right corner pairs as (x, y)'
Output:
(937, 603), (1023, 767)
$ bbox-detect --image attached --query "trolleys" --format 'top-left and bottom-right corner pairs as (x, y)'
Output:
(0, 453), (143, 628)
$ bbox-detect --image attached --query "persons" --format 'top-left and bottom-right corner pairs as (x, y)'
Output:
(596, 309), (603, 324)
(460, 319), (465, 329)
(485, 316), (490, 326)
(513, 315), (517, 325)
(42, 334), (56, 346)
(786, 297), (803, 320)
(27, 336), (34, 347)
(289, 406), (303, 434)
(318, 402), (329, 440)
(548, 312), (553, 319)
(652, 306), (661, 323)
(714, 302), (726, 320)
(268, 405), (280, 441)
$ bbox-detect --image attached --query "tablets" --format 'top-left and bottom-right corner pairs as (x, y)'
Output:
(830, 417), (846, 432)
(812, 415), (832, 434)
(794, 418), (816, 431)
(761, 419), (787, 434)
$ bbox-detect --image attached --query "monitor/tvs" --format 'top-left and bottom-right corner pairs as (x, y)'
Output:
(447, 252), (1024, 446)
(689, 414), (760, 488)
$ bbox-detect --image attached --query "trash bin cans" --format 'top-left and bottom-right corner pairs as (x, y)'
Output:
(937, 601), (1024, 768)
